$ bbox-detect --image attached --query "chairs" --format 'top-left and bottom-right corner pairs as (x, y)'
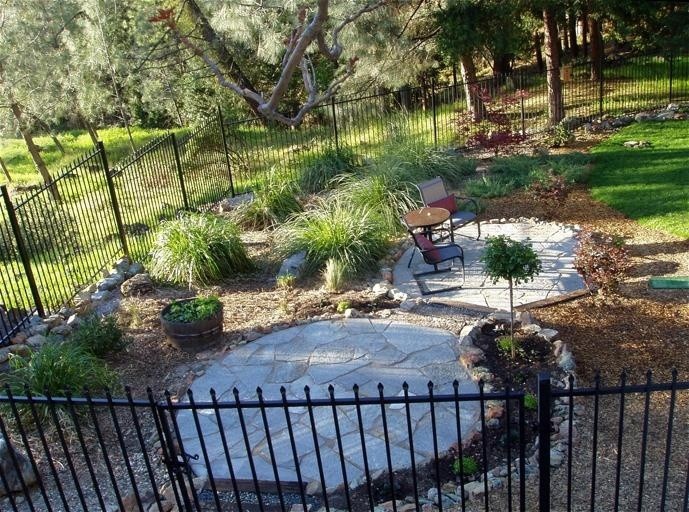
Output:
(406, 227), (465, 295)
(415, 175), (480, 243)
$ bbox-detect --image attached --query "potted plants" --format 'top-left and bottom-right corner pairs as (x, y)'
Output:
(159, 292), (224, 353)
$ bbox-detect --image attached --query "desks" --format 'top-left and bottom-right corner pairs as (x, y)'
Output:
(402, 207), (454, 265)
